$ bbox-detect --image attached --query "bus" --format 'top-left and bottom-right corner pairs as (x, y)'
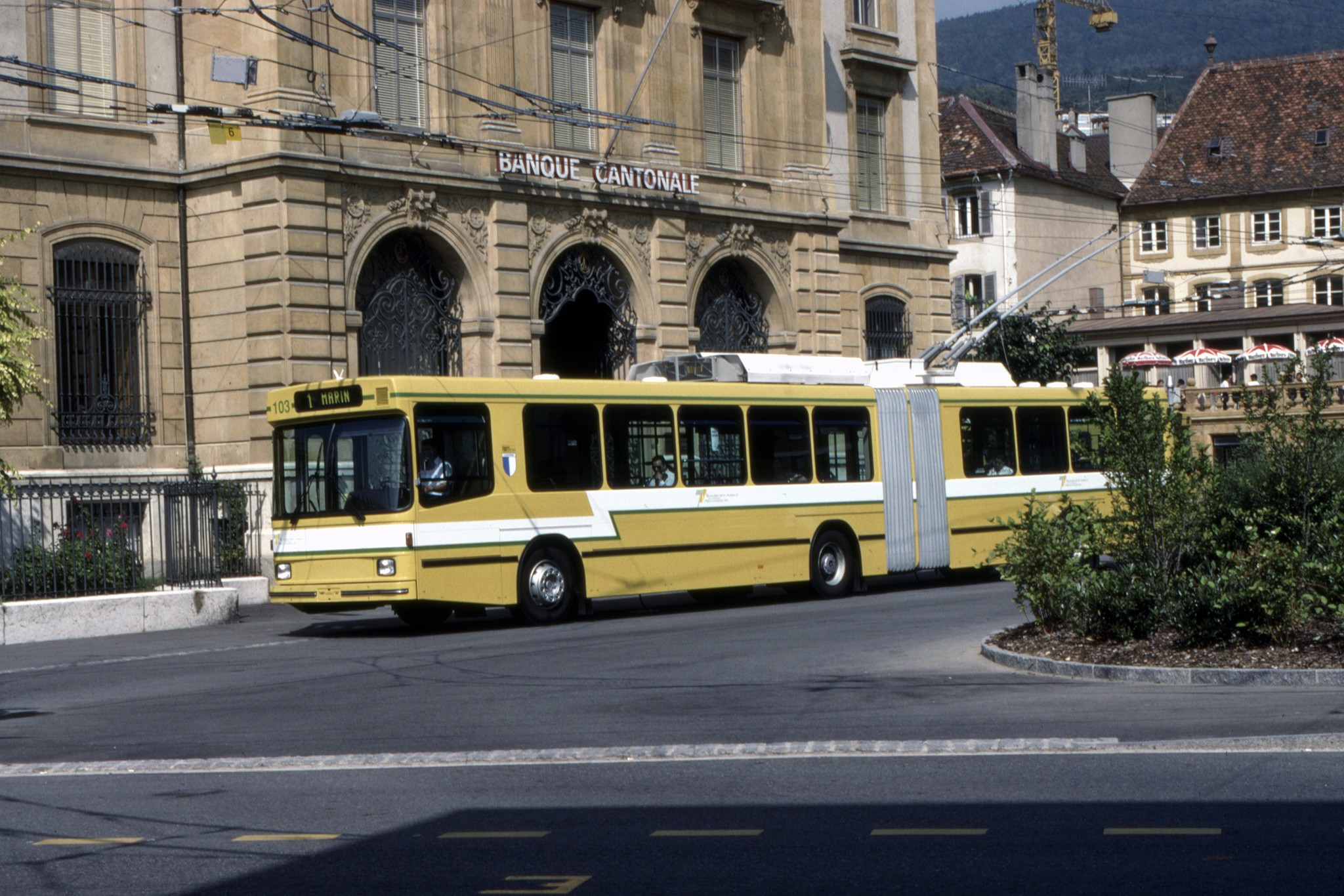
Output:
(266, 224), (1182, 628)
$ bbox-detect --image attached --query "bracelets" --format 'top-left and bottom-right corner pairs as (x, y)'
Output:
(430, 486), (434, 490)
(657, 477), (661, 480)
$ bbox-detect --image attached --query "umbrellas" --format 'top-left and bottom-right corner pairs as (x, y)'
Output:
(1235, 343), (1297, 375)
(1171, 348), (1232, 388)
(1305, 336), (1344, 357)
(1118, 351), (1172, 386)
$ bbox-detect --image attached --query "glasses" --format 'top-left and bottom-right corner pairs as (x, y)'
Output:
(652, 463), (662, 470)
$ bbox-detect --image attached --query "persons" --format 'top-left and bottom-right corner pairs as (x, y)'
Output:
(1278, 370), (1309, 401)
(1187, 378), (1195, 387)
(986, 454), (1014, 476)
(644, 455), (675, 487)
(420, 437), (453, 505)
(1176, 379), (1186, 388)
(1250, 374), (1263, 397)
(1219, 374), (1232, 401)
(1157, 380), (1164, 387)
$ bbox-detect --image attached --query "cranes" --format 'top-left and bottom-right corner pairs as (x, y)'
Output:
(1034, 0), (1118, 114)
